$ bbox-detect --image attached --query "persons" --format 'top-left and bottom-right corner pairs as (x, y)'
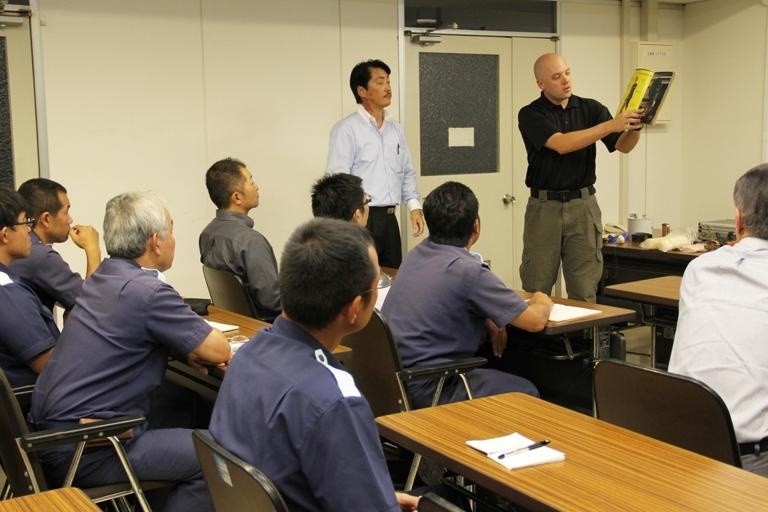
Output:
(378, 181), (553, 410)
(665, 160), (766, 478)
(516, 52), (648, 306)
(325, 58), (425, 270)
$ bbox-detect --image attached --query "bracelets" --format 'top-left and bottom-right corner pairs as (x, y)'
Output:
(629, 123), (642, 131)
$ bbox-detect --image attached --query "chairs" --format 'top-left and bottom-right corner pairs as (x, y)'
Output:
(0, 266), (768, 512)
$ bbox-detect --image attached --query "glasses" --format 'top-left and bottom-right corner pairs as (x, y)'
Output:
(359, 193), (373, 210)
(1, 217), (38, 227)
(359, 270), (394, 296)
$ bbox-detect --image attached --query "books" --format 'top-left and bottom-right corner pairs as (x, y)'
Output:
(523, 296), (602, 323)
(465, 430), (566, 472)
(612, 66), (673, 125)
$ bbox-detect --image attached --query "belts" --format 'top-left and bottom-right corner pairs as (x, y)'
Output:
(529, 186), (597, 202)
(737, 437), (768, 456)
(370, 206), (399, 214)
(25, 411), (82, 431)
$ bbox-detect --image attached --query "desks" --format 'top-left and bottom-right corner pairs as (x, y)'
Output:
(590, 228), (740, 265)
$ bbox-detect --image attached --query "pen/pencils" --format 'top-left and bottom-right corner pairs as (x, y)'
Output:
(498, 439), (551, 459)
